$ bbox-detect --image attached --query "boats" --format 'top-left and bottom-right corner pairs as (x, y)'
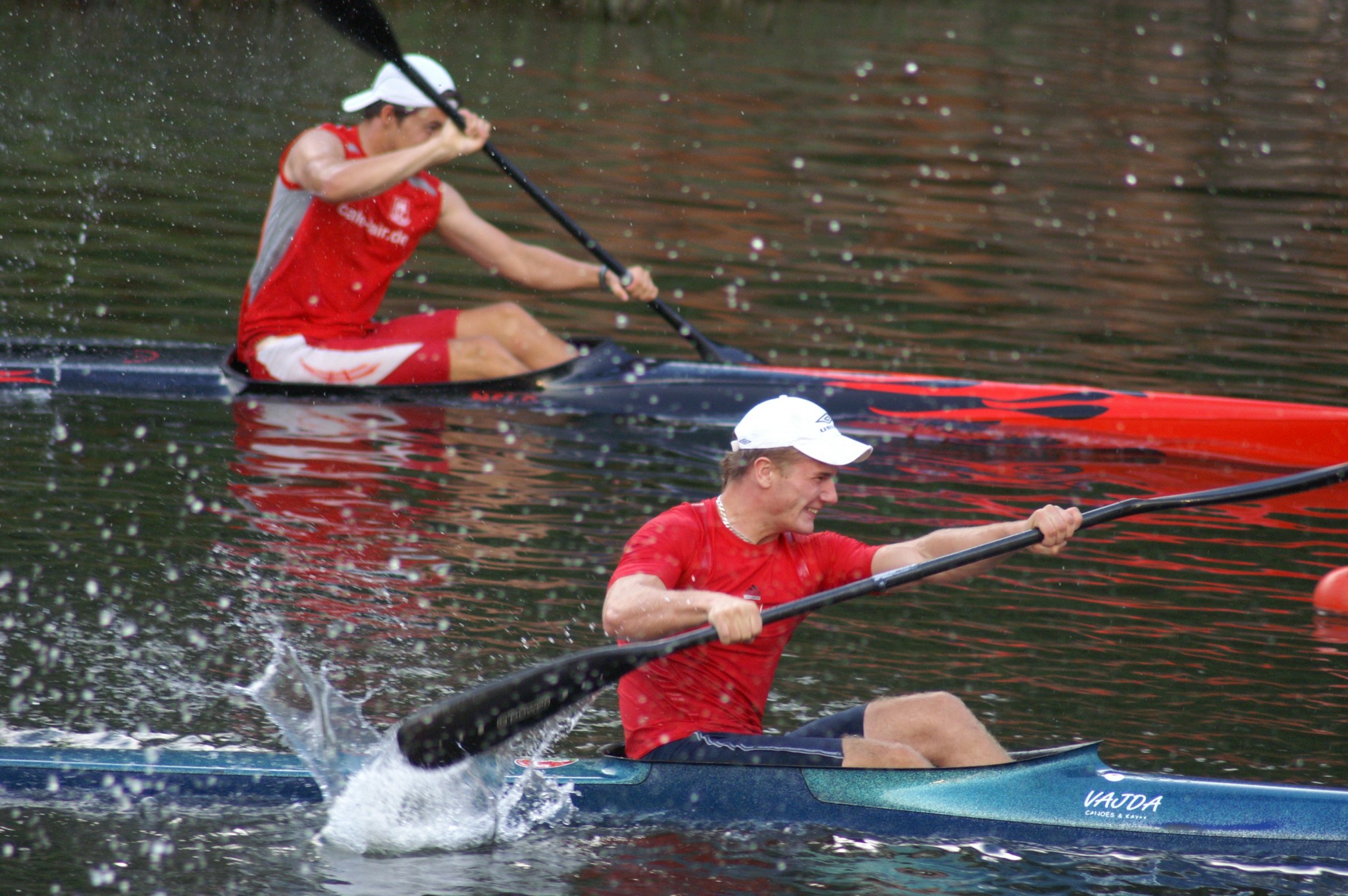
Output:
(0, 738), (1348, 857)
(0, 332), (1348, 469)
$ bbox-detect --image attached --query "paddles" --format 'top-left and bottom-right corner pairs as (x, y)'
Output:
(300, 0), (761, 368)
(396, 461), (1339, 772)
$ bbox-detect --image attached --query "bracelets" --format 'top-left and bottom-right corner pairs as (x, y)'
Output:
(599, 266), (611, 290)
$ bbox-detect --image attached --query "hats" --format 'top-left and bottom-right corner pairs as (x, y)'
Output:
(342, 54), (460, 114)
(731, 395), (873, 466)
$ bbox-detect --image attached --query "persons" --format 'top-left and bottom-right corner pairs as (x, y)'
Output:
(603, 393), (1082, 769)
(236, 53), (658, 384)
